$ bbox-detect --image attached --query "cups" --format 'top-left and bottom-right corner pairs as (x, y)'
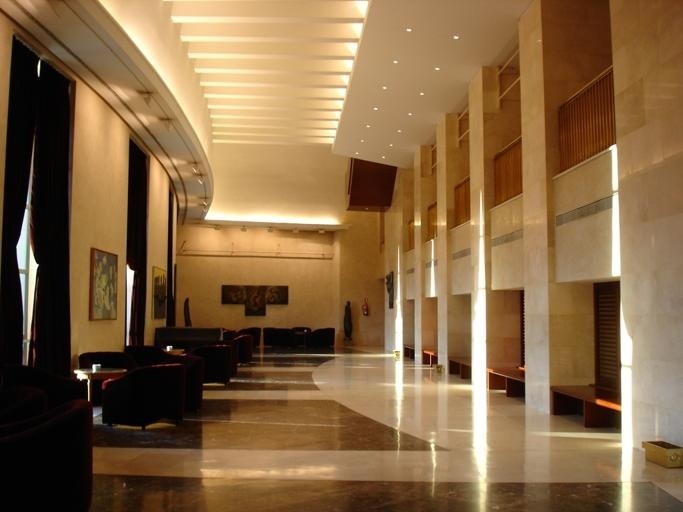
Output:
(92, 364), (102, 372)
(167, 345), (172, 351)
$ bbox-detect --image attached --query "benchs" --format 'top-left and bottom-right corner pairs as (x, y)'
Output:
(449, 357), (471, 378)
(423, 349), (438, 366)
(550, 385), (621, 427)
(486, 368), (525, 397)
(404, 345), (414, 359)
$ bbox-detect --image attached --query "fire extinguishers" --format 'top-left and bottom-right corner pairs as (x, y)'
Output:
(361, 296), (368, 316)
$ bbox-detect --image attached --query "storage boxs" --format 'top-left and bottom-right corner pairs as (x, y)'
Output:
(641, 441), (683, 468)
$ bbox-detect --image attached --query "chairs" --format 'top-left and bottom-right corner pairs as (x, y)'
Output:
(0, 327), (335, 512)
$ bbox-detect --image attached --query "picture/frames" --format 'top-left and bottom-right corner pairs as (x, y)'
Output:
(152, 266), (167, 320)
(88, 247), (118, 322)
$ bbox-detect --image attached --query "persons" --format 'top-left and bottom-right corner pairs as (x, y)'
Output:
(344, 300), (351, 340)
